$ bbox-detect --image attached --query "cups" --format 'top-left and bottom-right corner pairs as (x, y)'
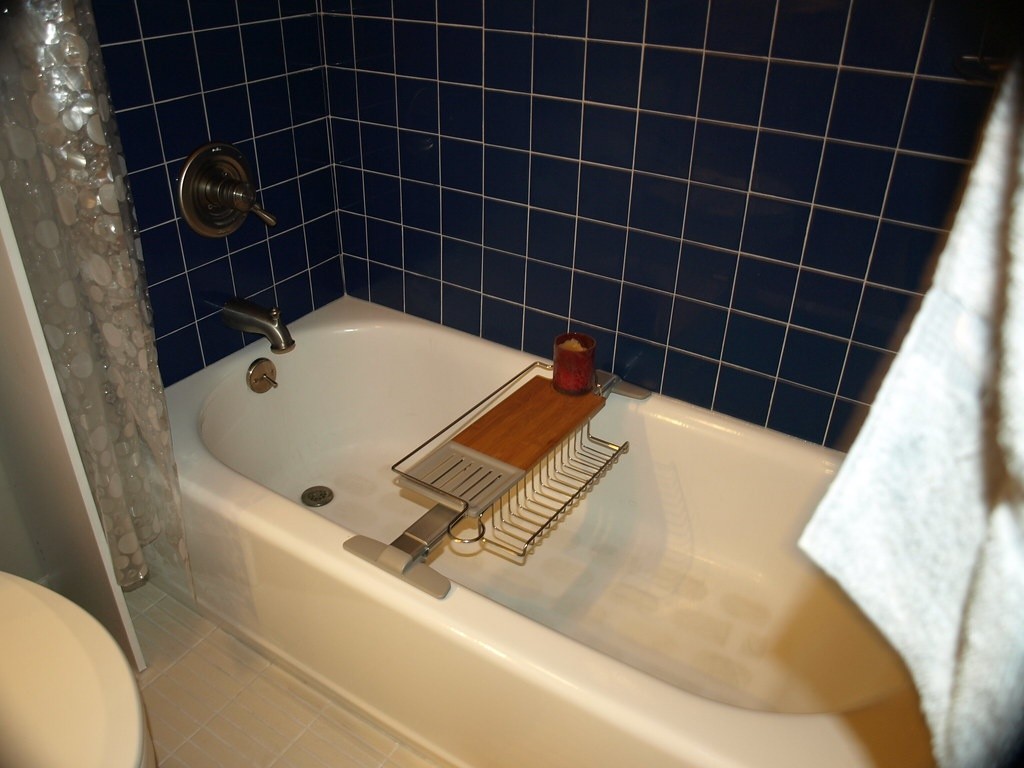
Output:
(552, 332), (597, 395)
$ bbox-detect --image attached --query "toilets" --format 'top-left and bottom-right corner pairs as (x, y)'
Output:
(3, 575), (141, 768)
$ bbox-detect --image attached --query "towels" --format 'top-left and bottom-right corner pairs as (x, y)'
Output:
(800, 65), (1024, 766)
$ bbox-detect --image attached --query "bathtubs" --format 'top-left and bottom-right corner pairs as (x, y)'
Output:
(161, 295), (933, 768)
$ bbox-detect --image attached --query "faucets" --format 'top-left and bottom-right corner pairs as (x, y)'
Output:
(220, 295), (296, 355)
